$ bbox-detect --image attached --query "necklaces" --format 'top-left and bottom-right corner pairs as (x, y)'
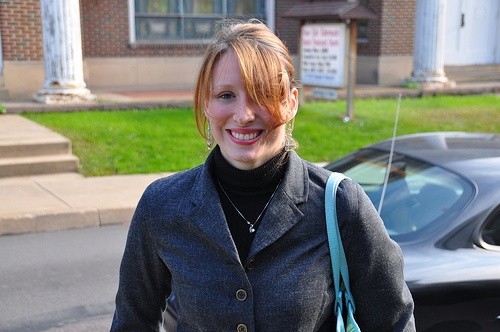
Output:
(215, 176), (283, 234)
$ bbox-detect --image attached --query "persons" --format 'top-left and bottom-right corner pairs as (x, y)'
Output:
(110, 21), (418, 332)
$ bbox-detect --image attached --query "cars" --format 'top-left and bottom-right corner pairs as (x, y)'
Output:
(322, 130), (500, 332)
